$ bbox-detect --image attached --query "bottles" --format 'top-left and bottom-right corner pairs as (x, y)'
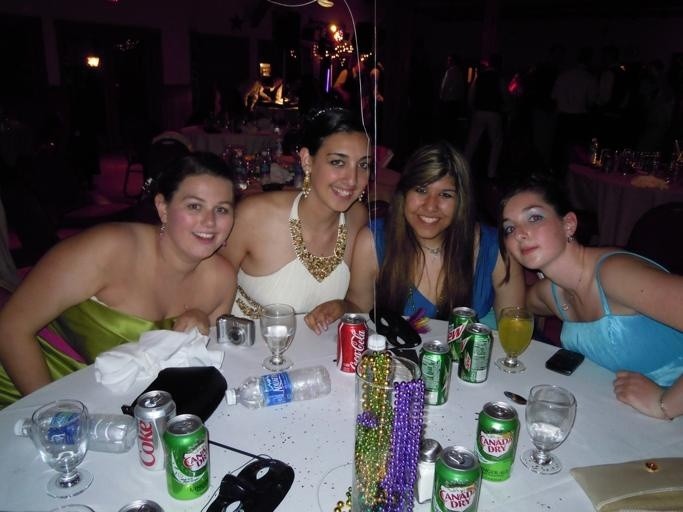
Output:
(13, 413), (138, 453)
(294, 158), (305, 190)
(226, 365), (332, 409)
(364, 336), (396, 385)
(261, 159), (271, 184)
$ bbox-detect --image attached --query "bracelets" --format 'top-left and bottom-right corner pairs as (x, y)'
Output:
(656, 386), (678, 422)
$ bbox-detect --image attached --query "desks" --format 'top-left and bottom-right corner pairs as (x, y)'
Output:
(0, 316), (682, 512)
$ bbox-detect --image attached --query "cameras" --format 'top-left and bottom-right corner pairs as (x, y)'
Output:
(216, 314), (256, 346)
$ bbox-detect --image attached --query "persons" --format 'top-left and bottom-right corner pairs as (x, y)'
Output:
(302, 143), (523, 336)
(499, 184), (683, 424)
(462, 53), (515, 175)
(207, 107), (374, 320)
(439, 53), (464, 139)
(0, 152), (242, 405)
(359, 48), (388, 128)
(553, 63), (613, 141)
(313, 18), (358, 110)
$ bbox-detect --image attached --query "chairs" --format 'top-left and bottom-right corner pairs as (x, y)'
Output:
(144, 138), (190, 201)
(122, 127), (149, 200)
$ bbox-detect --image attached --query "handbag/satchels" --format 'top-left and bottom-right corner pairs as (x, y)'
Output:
(121, 366), (227, 425)
(571, 458), (683, 512)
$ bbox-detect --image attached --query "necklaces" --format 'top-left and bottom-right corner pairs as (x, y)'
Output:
(556, 248), (596, 311)
(417, 238), (447, 255)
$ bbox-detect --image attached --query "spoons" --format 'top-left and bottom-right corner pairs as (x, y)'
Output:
(504, 390), (577, 407)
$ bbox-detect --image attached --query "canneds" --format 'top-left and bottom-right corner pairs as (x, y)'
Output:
(477, 400), (521, 481)
(336, 313), (370, 375)
(418, 341), (455, 407)
(162, 413), (210, 501)
(447, 306), (479, 363)
(132, 389), (178, 472)
(457, 322), (494, 383)
(430, 446), (484, 512)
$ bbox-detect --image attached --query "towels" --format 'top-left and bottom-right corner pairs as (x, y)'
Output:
(93, 327), (225, 398)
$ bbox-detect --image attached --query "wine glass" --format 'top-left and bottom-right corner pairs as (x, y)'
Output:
(31, 398), (95, 498)
(494, 307), (535, 374)
(521, 384), (577, 476)
(261, 303), (297, 371)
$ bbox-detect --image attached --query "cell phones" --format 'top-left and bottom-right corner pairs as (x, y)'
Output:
(545, 348), (585, 376)
